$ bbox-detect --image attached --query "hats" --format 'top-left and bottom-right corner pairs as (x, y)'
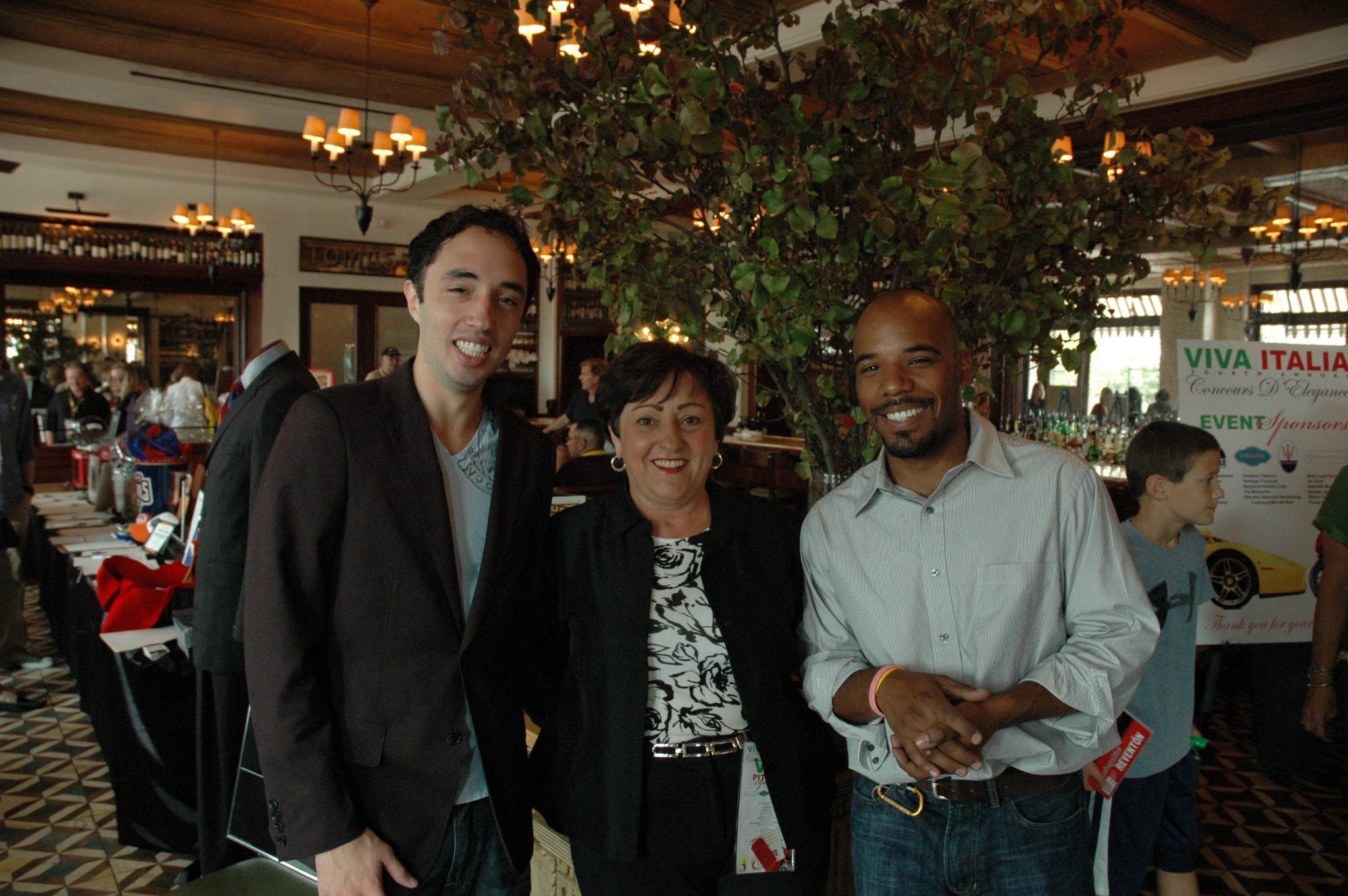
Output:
(381, 346), (403, 356)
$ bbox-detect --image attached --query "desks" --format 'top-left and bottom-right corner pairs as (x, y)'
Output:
(23, 488), (222, 874)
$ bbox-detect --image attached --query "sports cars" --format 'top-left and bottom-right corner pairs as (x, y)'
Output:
(1192, 526), (1308, 610)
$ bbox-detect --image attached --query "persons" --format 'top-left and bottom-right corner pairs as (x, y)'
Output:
(1298, 460), (1348, 745)
(523, 342), (841, 896)
(536, 356), (627, 487)
(21, 357), (217, 457)
(1109, 415), (1224, 896)
(238, 203), (559, 896)
(1029, 378), (1171, 429)
(193, 340), (322, 878)
(0, 364), (56, 713)
(363, 346), (402, 381)
(801, 282), (1162, 896)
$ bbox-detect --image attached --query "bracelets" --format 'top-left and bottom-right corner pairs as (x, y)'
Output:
(1306, 665), (1334, 688)
(867, 663), (906, 718)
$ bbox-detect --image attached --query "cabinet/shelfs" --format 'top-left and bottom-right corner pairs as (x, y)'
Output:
(511, 267), (609, 352)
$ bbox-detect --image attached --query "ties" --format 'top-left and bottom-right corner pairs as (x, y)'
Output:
(217, 375), (243, 426)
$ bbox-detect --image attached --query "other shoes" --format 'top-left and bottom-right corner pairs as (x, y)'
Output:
(12, 652), (54, 669)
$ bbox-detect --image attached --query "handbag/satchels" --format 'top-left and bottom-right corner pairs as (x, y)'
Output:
(95, 554), (196, 633)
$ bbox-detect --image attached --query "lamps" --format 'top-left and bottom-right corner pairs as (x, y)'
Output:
(1220, 250), (1274, 339)
(172, 130), (255, 283)
(1162, 259), (1226, 324)
(1249, 129), (1348, 293)
(693, 201), (774, 236)
(302, 0), (427, 237)
(1051, 129), (1152, 181)
(529, 236), (581, 302)
(37, 286), (116, 319)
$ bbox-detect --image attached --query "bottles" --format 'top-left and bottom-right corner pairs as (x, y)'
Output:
(0, 218), (261, 267)
(566, 298), (608, 320)
(999, 408), (1151, 465)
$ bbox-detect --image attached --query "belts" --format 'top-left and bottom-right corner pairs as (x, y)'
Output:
(910, 771), (1066, 804)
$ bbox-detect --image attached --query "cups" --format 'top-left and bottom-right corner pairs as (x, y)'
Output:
(111, 468), (136, 536)
